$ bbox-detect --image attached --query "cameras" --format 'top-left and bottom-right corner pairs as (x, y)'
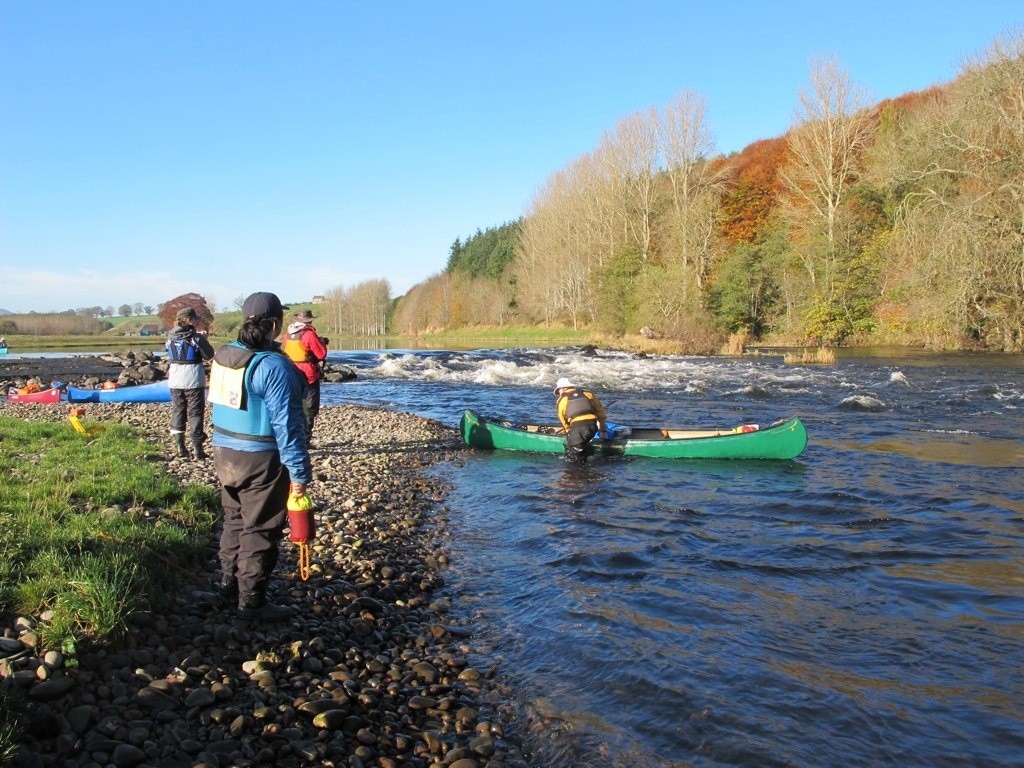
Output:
(319, 337), (329, 345)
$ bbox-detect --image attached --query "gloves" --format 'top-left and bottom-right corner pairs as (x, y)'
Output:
(323, 338), (329, 345)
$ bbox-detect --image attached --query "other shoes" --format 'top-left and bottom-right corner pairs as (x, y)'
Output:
(220, 579), (239, 602)
(238, 601), (292, 621)
(306, 441), (317, 450)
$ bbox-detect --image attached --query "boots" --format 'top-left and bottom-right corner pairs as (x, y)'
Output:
(173, 433), (189, 457)
(192, 441), (210, 460)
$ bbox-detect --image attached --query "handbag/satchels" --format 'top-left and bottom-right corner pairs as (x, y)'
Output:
(308, 351), (319, 363)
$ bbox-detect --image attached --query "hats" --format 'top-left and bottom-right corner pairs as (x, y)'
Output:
(292, 310), (318, 323)
(554, 378), (577, 394)
(243, 292), (290, 322)
(177, 309), (201, 320)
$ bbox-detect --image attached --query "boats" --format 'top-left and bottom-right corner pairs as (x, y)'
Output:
(7, 385), (61, 404)
(459, 407), (809, 459)
(67, 378), (172, 403)
(0, 347), (8, 355)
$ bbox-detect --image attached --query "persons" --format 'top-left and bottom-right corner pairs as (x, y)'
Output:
(553, 378), (607, 465)
(206, 291), (313, 622)
(165, 308), (214, 459)
(281, 308), (330, 449)
